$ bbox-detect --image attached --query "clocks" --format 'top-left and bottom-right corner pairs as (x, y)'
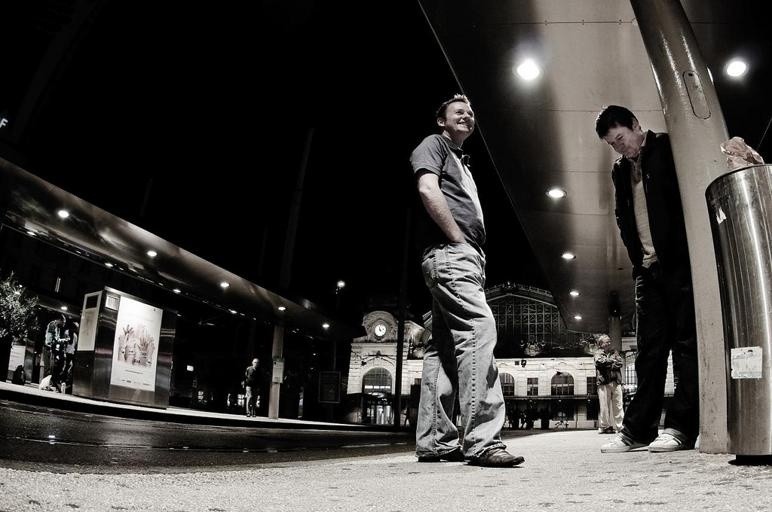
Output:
(375, 324), (387, 337)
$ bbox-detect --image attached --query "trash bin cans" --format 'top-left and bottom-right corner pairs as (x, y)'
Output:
(704, 165), (772, 465)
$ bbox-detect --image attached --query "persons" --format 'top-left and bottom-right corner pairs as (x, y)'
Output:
(242, 357), (266, 417)
(45, 313), (78, 393)
(594, 335), (625, 434)
(596, 105), (694, 452)
(410, 93), (524, 467)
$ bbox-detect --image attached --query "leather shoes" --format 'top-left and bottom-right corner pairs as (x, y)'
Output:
(467, 450), (525, 465)
(418, 448), (464, 463)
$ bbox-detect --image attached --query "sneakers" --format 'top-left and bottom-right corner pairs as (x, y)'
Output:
(601, 434), (646, 454)
(648, 432), (685, 452)
